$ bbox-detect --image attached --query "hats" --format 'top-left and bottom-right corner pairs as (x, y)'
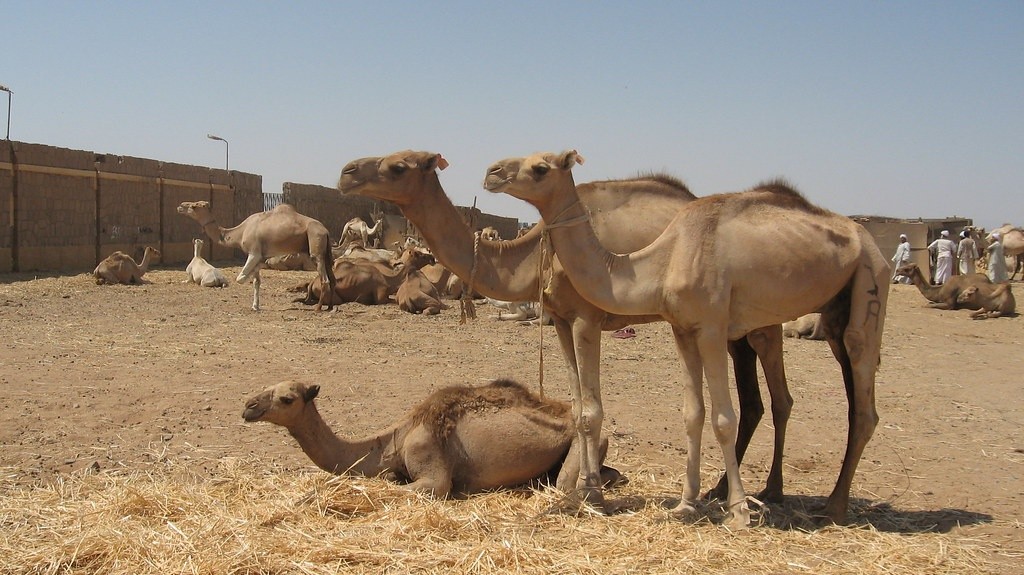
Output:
(960, 231), (965, 237)
(992, 233), (1000, 240)
(900, 234), (908, 242)
(941, 230), (949, 236)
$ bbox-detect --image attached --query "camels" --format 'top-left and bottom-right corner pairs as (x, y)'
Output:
(895, 261), (1016, 319)
(242, 381), (620, 501)
(176, 200), (588, 328)
(92, 246), (161, 285)
(963, 224), (1024, 284)
(338, 147), (890, 532)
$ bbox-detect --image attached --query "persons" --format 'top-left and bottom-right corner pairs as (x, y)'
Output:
(985, 233), (1010, 284)
(928, 230), (956, 286)
(957, 230), (979, 275)
(890, 234), (915, 285)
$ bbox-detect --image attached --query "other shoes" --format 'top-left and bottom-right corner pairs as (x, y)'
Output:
(910, 283), (914, 285)
(893, 281), (899, 284)
(936, 282), (941, 285)
